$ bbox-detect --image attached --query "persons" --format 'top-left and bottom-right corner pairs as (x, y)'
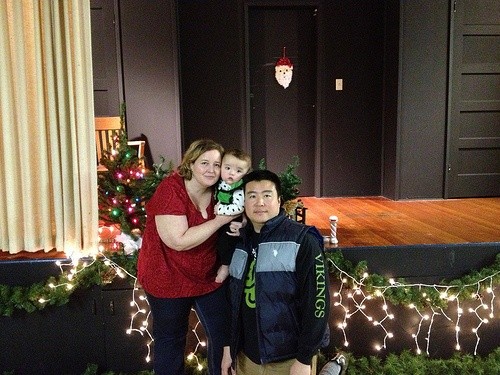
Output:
(214, 149), (253, 283)
(137, 136), (247, 375)
(221, 169), (330, 375)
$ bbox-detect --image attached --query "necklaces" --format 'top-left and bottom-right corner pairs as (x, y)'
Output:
(250, 243), (258, 257)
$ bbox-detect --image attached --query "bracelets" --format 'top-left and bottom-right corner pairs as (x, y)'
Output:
(214, 219), (221, 227)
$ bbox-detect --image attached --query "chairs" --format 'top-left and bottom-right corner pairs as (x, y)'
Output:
(95, 116), (124, 174)
